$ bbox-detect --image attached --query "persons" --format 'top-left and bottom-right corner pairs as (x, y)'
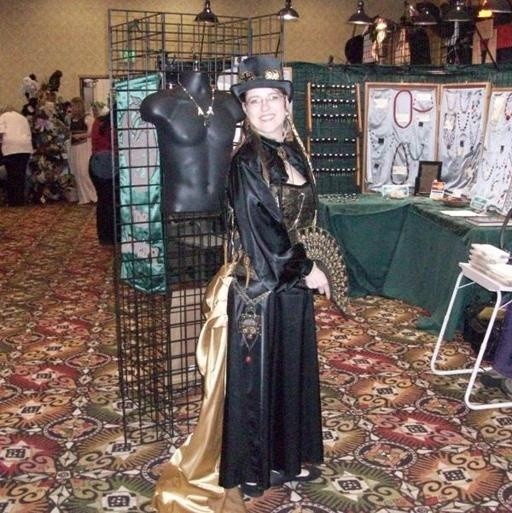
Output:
(221, 56), (331, 497)
(0, 92), (109, 204)
(140, 71), (246, 209)
(88, 102), (120, 244)
(483, 295), (512, 399)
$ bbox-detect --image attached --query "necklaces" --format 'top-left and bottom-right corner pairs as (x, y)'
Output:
(371, 85), (512, 213)
(174, 78), (216, 125)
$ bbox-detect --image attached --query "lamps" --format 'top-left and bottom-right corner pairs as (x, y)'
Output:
(482, 0), (512, 15)
(413, 0), (439, 25)
(349, 0), (375, 24)
(444, 0), (470, 22)
(194, 0), (219, 23)
(275, 0), (299, 21)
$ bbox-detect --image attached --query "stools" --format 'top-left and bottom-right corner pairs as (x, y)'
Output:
(431, 261), (512, 410)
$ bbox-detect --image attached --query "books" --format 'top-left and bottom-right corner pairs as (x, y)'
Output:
(466, 216), (512, 227)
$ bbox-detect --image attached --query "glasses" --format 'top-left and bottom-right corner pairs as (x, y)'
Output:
(242, 91), (284, 108)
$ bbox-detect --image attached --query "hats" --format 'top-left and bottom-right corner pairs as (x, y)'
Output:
(231, 55), (294, 104)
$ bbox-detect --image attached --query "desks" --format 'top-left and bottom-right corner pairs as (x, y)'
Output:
(316, 186), (512, 342)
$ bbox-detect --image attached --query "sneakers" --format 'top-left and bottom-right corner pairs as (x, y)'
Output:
(269, 466), (322, 489)
(241, 480), (263, 497)
